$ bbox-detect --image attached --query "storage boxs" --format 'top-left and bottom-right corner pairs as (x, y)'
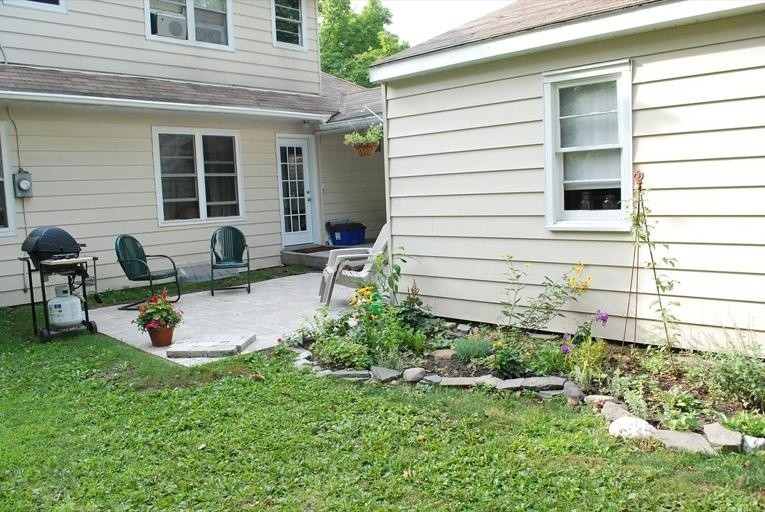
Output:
(329, 223), (367, 245)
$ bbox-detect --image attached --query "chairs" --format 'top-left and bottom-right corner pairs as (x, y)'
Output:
(116, 234), (180, 310)
(210, 226), (250, 296)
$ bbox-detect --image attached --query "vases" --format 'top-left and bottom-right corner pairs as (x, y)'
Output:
(147, 326), (173, 346)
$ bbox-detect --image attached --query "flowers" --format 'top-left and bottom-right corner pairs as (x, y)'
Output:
(131, 287), (185, 335)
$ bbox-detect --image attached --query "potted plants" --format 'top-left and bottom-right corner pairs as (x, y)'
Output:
(344, 122), (383, 158)
